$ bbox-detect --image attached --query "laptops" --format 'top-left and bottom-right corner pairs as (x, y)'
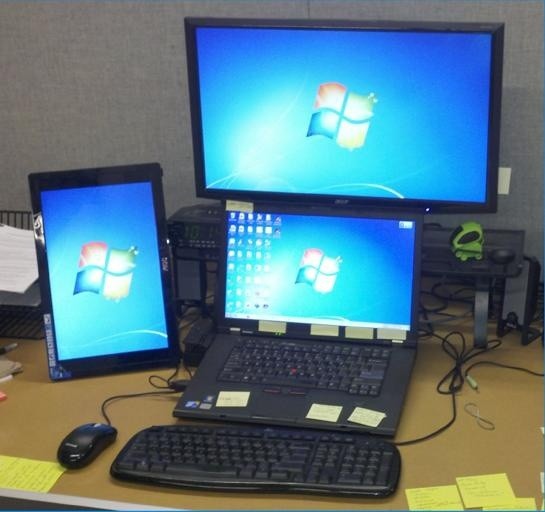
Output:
(173, 196), (423, 436)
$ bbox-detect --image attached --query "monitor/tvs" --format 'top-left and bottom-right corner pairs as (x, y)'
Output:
(184, 16), (506, 213)
(28, 162), (180, 381)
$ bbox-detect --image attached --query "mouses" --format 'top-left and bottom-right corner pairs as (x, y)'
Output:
(57, 422), (117, 471)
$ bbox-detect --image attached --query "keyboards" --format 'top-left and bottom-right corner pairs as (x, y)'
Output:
(109, 423), (402, 499)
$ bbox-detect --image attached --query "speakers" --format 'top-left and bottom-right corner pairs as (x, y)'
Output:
(176, 259), (209, 318)
(496, 255), (541, 346)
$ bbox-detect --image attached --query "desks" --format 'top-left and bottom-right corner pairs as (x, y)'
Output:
(0, 283), (545, 512)
